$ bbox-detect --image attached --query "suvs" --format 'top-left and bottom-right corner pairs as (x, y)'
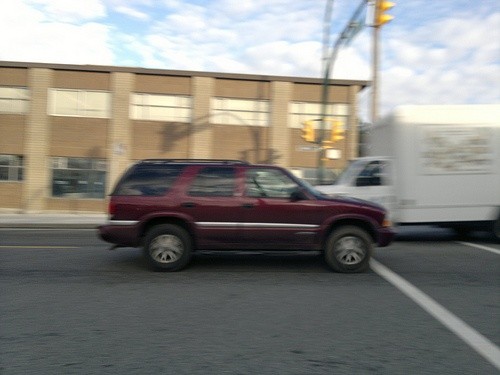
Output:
(94, 158), (398, 275)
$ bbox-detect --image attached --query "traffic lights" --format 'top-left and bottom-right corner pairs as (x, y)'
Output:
(364, 0), (395, 29)
(330, 119), (347, 143)
(298, 120), (316, 143)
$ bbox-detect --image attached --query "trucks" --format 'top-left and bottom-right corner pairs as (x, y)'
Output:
(326, 102), (500, 244)
